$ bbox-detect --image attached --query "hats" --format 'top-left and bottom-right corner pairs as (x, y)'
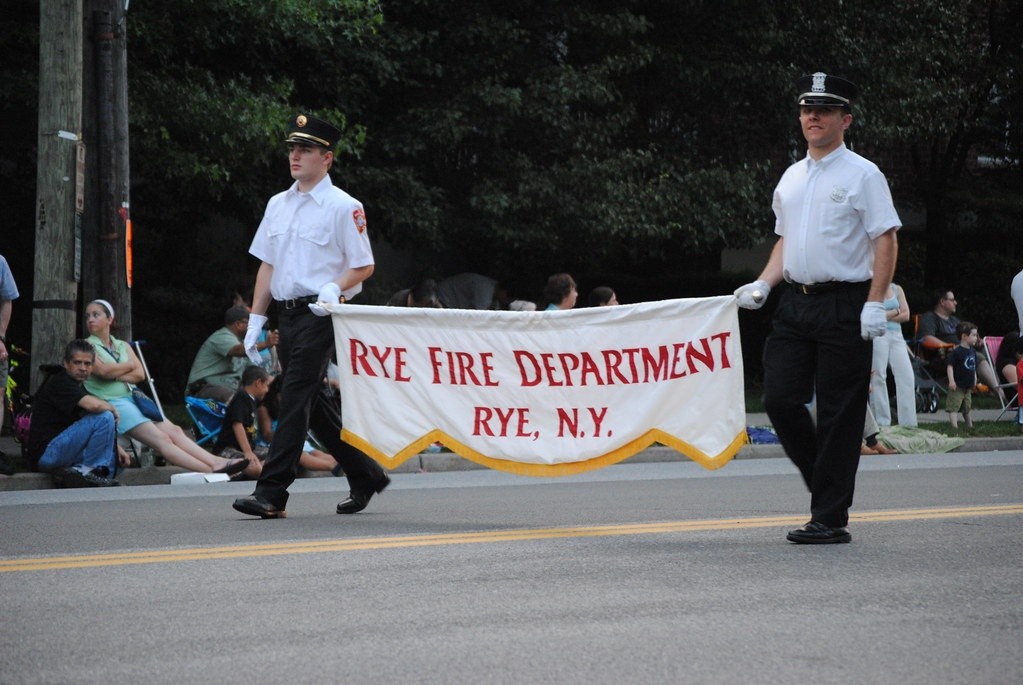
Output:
(284, 113), (342, 149)
(796, 71), (859, 106)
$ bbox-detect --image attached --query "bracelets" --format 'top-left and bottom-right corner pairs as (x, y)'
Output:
(0, 335), (6, 344)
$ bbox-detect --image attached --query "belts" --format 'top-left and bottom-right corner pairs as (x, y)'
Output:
(274, 294), (319, 310)
(782, 278), (872, 295)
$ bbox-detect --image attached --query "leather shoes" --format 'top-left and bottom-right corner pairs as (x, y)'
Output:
(860, 442), (898, 454)
(232, 494), (287, 519)
(787, 519), (852, 542)
(336, 470), (391, 513)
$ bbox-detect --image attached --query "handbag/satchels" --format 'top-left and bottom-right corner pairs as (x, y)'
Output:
(132, 388), (163, 421)
(14, 408), (33, 445)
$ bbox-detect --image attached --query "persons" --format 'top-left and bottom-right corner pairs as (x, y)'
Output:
(732, 73), (903, 544)
(0, 250), (1023, 486)
(231, 111), (393, 519)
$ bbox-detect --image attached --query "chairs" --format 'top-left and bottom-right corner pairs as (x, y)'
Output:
(982, 336), (1017, 423)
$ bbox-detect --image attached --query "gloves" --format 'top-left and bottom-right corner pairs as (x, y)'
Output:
(733, 280), (771, 309)
(243, 313), (268, 366)
(308, 281), (341, 315)
(860, 300), (888, 341)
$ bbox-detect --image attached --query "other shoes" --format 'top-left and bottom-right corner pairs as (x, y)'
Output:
(331, 463), (345, 476)
(53, 465), (119, 486)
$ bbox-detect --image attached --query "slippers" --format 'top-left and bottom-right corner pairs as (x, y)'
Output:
(213, 457), (250, 476)
